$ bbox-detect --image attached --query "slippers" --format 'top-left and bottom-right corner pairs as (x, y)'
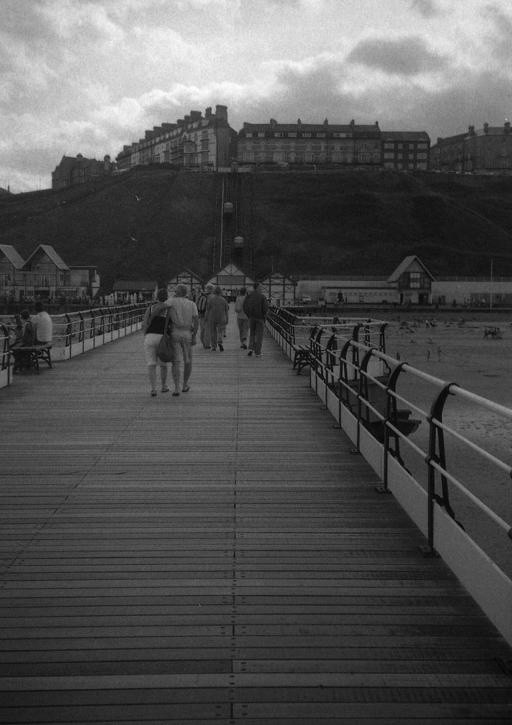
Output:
(150, 386), (189, 396)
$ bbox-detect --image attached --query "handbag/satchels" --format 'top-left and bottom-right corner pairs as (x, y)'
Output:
(158, 334), (176, 362)
(235, 295), (242, 312)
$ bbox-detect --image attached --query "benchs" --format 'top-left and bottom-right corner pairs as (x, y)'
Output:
(291, 342), (324, 375)
(12, 342), (53, 375)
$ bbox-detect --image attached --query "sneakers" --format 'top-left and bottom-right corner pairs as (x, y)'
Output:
(239, 343), (248, 350)
(248, 350), (265, 357)
(204, 344), (224, 352)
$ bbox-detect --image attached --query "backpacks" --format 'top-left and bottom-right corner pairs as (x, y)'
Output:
(196, 292), (211, 317)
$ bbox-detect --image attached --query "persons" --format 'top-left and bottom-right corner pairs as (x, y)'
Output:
(9, 311), (34, 370)
(28, 301), (53, 367)
(146, 284), (199, 396)
(190, 282), (269, 358)
(144, 289), (172, 396)
